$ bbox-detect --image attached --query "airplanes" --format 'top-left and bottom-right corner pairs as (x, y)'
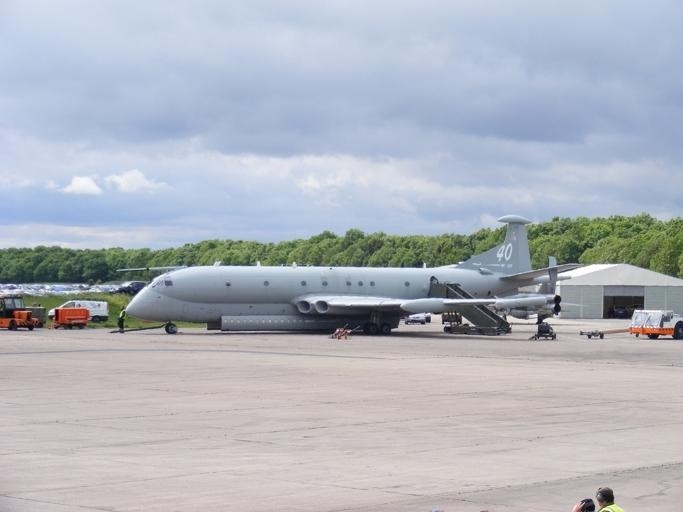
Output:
(116, 214), (584, 336)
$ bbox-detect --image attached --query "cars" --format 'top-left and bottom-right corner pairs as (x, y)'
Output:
(405, 312), (431, 325)
(109, 281), (147, 296)
(609, 304), (644, 318)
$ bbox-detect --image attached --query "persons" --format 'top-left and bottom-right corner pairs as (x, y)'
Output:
(116, 305), (127, 333)
(571, 497), (596, 511)
(595, 485), (625, 512)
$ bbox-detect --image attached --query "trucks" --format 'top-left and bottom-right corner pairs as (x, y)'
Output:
(630, 310), (683, 339)
(0, 295), (40, 331)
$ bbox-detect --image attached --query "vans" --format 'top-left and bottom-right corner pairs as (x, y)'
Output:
(48, 300), (108, 323)
